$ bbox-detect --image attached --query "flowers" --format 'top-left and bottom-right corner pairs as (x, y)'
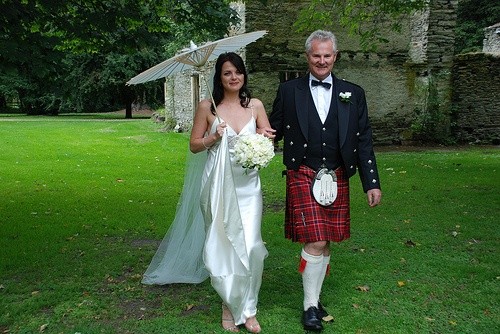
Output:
(338, 91), (354, 103)
(233, 134), (274, 172)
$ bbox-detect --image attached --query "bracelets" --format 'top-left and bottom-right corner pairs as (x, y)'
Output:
(202, 137), (213, 149)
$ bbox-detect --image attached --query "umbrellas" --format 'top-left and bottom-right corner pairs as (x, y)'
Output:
(126, 29), (269, 124)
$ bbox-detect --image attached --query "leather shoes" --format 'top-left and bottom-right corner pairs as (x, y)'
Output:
(317, 302), (334, 322)
(301, 306), (323, 333)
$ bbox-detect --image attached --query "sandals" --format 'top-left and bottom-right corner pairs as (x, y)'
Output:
(222, 302), (241, 332)
(244, 316), (261, 334)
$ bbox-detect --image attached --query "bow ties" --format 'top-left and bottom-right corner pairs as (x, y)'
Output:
(311, 80), (331, 89)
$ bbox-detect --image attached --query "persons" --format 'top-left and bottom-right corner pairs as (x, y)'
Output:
(189, 51), (274, 333)
(256, 30), (382, 333)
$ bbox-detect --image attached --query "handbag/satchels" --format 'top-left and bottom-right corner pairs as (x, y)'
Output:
(311, 168), (338, 206)
